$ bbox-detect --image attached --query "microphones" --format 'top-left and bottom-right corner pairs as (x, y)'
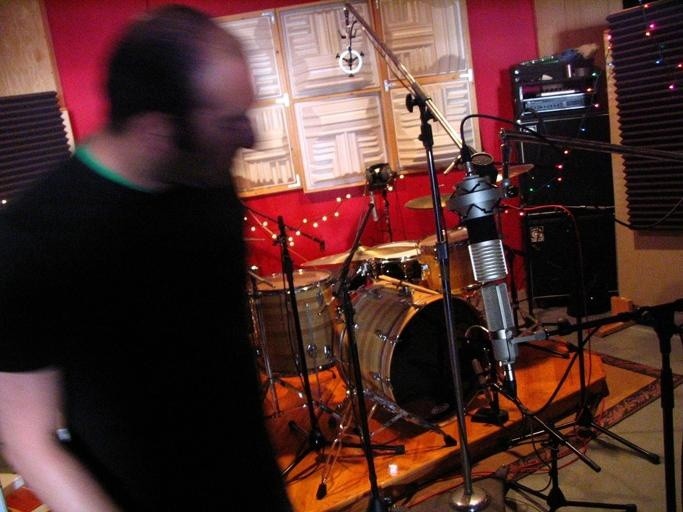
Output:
(448, 177), (519, 366)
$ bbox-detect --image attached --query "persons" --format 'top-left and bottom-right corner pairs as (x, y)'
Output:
(0, 0), (300, 511)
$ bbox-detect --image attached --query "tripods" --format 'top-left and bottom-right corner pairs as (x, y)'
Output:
(316, 297), (456, 501)
(506, 432), (638, 512)
(501, 317), (661, 466)
(269, 230), (406, 479)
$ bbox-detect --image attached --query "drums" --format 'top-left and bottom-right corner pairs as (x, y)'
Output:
(252, 269), (341, 377)
(329, 274), (492, 430)
(421, 229), (478, 300)
(371, 239), (422, 287)
(304, 250), (357, 292)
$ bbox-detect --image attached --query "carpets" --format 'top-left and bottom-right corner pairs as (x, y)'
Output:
(389, 349), (683, 512)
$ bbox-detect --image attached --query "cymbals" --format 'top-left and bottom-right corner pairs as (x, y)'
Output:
(497, 164), (534, 183)
(404, 194), (450, 209)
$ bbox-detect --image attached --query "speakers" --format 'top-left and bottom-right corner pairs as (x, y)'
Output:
(517, 108), (618, 316)
